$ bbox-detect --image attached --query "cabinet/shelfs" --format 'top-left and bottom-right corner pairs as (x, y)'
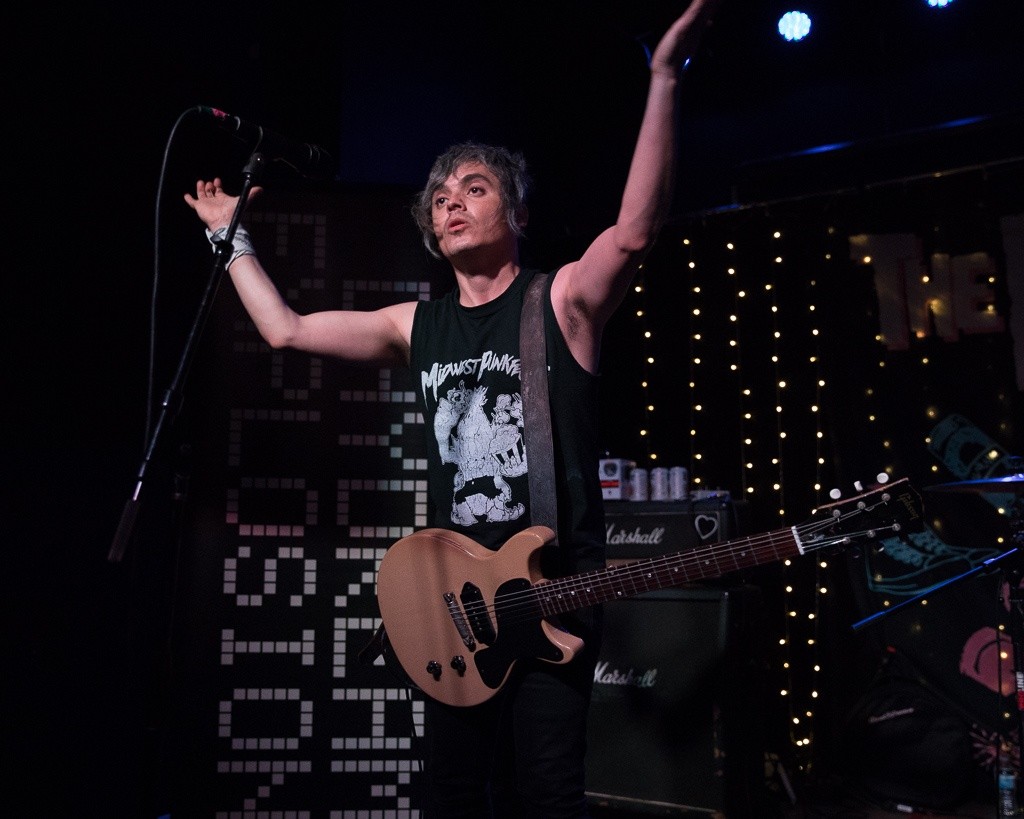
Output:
(583, 501), (736, 819)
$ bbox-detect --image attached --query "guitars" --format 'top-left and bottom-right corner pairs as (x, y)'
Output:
(377, 476), (933, 708)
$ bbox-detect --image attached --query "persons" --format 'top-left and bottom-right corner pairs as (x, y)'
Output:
(183, 0), (703, 819)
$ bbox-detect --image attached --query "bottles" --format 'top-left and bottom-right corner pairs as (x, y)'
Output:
(998, 775), (1020, 818)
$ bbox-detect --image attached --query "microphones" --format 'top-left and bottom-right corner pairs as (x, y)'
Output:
(220, 112), (326, 177)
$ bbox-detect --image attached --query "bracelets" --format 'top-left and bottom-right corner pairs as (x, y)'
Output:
(204, 223), (255, 272)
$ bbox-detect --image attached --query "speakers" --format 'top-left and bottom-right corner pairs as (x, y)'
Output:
(583, 587), (776, 819)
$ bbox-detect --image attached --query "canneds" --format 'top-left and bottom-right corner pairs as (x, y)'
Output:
(650, 466), (689, 501)
(629, 468), (649, 501)
